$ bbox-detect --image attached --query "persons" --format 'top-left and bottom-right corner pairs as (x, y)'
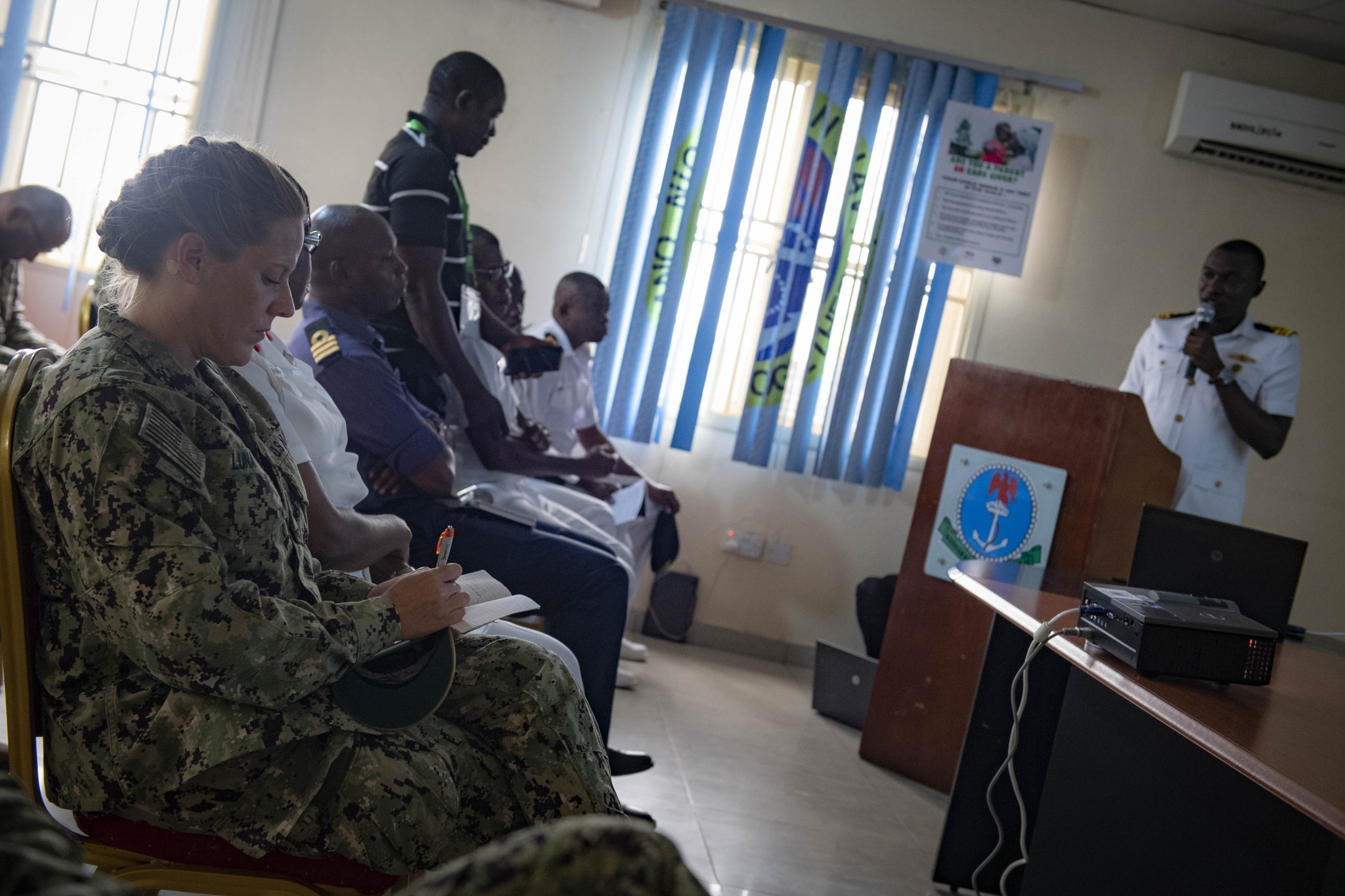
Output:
(1, 49), (713, 896)
(981, 122), (1039, 169)
(1117, 238), (1301, 524)
(10, 136), (620, 877)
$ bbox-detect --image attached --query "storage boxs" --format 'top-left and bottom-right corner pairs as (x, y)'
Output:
(810, 639), (879, 729)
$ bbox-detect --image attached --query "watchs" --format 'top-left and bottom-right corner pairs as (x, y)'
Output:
(1209, 365), (1234, 386)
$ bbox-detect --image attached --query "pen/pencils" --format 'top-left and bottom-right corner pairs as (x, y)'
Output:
(435, 525), (455, 568)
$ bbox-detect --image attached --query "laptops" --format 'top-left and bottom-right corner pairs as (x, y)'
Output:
(1126, 502), (1308, 644)
(813, 639), (879, 731)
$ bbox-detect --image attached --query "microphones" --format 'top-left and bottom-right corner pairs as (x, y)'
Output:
(1185, 301), (1217, 380)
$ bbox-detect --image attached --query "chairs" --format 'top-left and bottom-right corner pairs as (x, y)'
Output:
(0, 285), (544, 896)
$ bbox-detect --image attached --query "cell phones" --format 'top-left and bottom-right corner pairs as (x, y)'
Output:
(502, 347), (564, 375)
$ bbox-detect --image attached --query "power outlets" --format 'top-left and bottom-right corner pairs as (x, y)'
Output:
(723, 528), (767, 560)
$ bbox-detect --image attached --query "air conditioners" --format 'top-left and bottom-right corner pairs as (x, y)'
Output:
(1162, 68), (1344, 194)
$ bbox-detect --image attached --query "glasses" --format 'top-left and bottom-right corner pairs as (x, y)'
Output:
(473, 261), (514, 284)
(305, 230), (323, 254)
(28, 215), (52, 254)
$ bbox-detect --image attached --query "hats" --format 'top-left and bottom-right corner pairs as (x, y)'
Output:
(323, 628), (457, 733)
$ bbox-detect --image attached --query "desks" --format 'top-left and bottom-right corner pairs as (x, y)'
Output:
(931, 561), (1345, 896)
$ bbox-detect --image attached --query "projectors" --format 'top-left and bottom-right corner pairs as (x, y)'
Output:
(1077, 581), (1278, 685)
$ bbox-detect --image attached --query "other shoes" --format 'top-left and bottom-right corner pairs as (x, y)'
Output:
(621, 803), (657, 832)
(616, 668), (638, 688)
(605, 747), (653, 775)
(619, 637), (649, 661)
